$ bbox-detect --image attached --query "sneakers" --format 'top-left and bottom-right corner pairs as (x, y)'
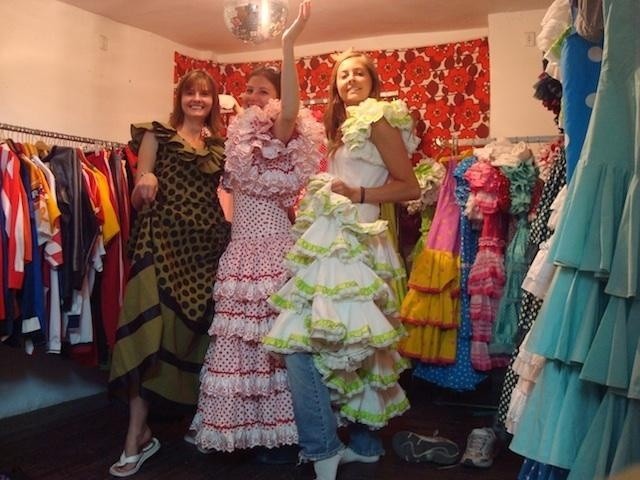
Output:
(461, 427), (501, 467)
(392, 430), (460, 464)
(261, 447), (298, 464)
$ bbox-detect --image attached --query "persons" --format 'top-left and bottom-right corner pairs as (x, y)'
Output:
(260, 48), (424, 480)
(106, 68), (233, 476)
(186, 1), (326, 464)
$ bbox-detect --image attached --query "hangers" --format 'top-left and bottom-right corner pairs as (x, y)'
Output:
(0, 120), (131, 160)
(448, 133), (545, 162)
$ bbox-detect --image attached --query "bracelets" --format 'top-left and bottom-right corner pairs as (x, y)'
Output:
(139, 171), (151, 178)
(357, 184), (367, 203)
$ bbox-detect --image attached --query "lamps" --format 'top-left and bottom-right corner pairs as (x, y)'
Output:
(220, 0), (289, 48)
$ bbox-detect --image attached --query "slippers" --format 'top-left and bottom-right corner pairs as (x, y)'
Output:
(110, 437), (161, 477)
